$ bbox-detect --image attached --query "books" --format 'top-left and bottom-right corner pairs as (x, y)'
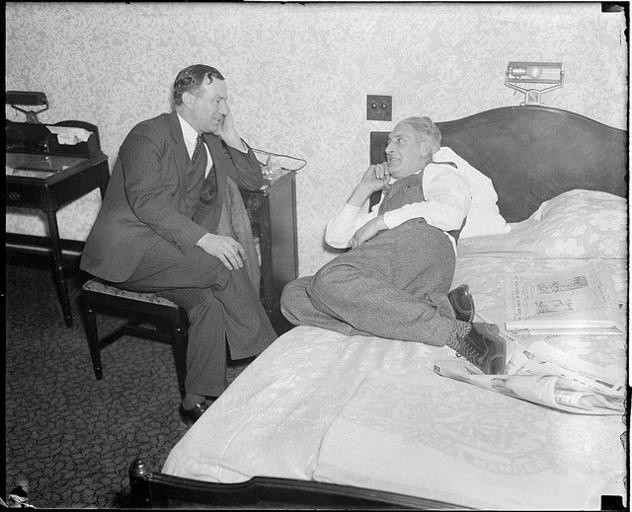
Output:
(504, 260), (625, 336)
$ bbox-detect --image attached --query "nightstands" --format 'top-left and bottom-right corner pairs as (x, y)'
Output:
(238, 165), (299, 324)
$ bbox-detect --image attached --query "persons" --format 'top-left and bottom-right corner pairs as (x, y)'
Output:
(77, 63), (280, 426)
(280, 115), (507, 382)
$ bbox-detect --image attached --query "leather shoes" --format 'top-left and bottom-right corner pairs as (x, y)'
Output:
(178, 402), (209, 428)
(448, 283), (475, 324)
(458, 320), (507, 377)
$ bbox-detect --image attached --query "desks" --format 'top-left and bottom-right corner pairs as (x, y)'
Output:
(5, 153), (110, 328)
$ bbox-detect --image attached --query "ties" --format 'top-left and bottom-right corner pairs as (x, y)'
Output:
(190, 134), (208, 182)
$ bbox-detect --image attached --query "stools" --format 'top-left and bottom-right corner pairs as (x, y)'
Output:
(78, 279), (189, 400)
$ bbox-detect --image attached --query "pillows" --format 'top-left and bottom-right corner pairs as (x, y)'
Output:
(431, 147), (509, 239)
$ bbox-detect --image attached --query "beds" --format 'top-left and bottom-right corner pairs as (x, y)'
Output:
(129, 104), (628, 512)
